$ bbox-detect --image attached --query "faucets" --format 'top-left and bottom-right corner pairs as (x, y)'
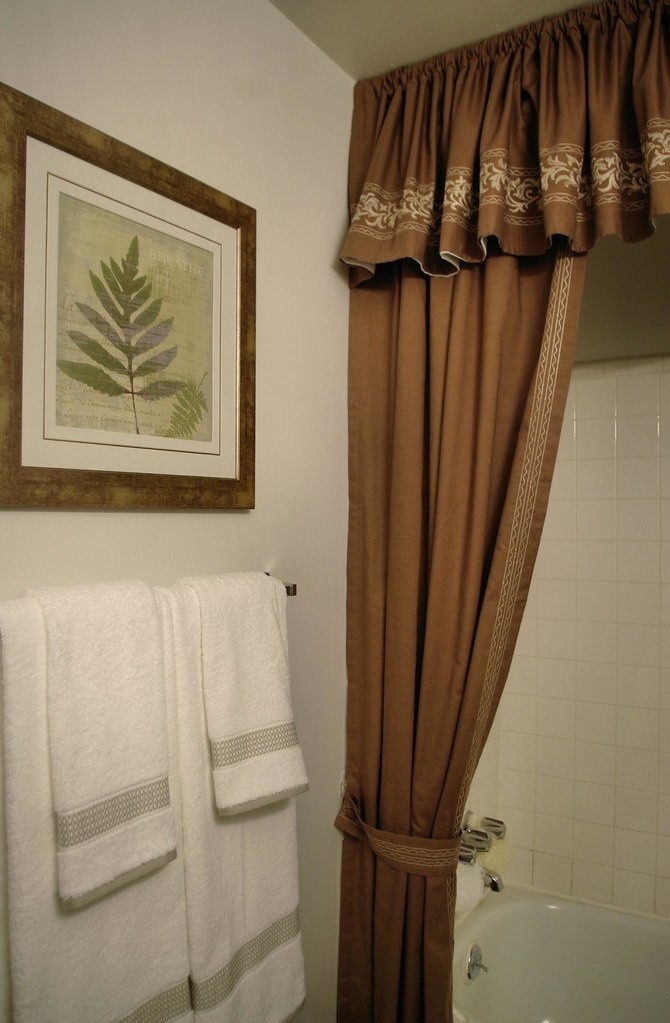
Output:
(481, 865), (505, 893)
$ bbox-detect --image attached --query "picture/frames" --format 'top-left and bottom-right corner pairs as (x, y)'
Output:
(0, 77), (257, 509)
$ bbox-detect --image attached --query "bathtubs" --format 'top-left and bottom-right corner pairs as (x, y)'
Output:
(451, 885), (670, 1022)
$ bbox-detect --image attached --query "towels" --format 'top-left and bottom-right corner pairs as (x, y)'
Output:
(161, 570), (305, 1020)
(1, 581), (197, 1022)
